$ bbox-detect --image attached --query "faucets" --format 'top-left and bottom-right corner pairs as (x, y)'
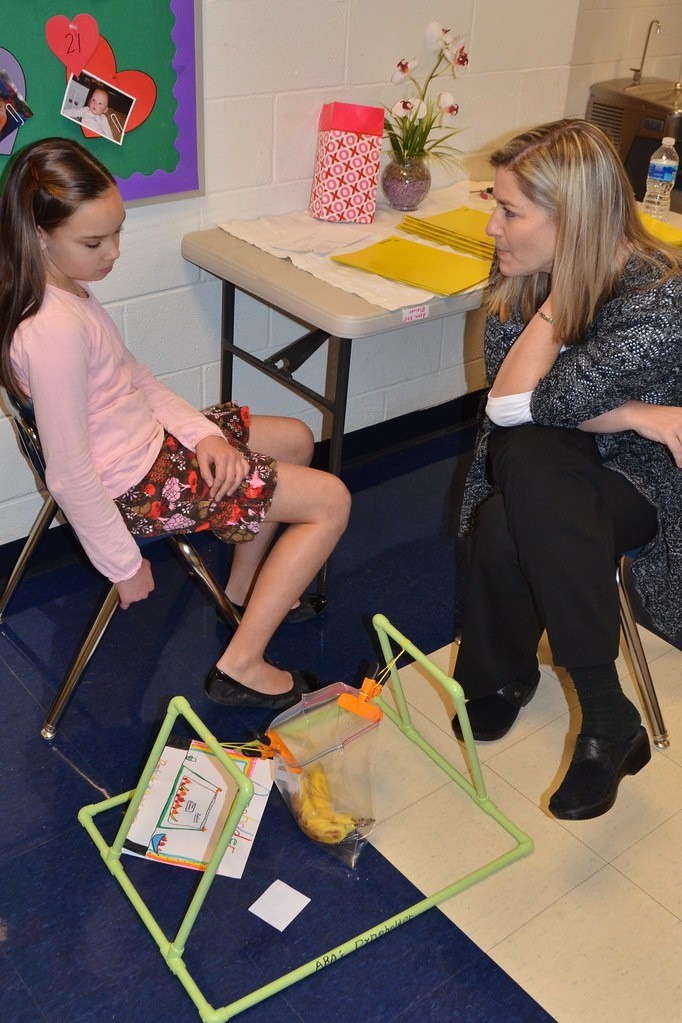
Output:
(629, 19), (661, 85)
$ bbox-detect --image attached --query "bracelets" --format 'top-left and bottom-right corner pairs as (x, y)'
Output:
(538, 309), (553, 325)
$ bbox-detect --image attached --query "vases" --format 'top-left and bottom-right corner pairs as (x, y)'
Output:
(382, 156), (431, 212)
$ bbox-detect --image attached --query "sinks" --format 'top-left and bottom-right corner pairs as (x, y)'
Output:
(625, 82), (682, 108)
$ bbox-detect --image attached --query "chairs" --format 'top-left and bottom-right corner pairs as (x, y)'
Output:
(0, 387), (283, 739)
(455, 544), (670, 750)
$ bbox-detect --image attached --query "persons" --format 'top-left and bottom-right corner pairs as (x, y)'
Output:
(0, 137), (352, 709)
(0, 79), (26, 138)
(450, 119), (682, 822)
(65, 89), (114, 140)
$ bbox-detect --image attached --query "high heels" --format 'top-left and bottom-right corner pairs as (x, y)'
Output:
(452, 654), (540, 741)
(548, 726), (651, 821)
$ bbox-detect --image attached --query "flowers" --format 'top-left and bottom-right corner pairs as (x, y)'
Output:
(377, 15), (481, 171)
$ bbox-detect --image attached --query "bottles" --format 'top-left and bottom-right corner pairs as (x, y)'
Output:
(642, 136), (679, 221)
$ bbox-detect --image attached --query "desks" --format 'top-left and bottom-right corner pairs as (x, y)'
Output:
(183, 180), (682, 614)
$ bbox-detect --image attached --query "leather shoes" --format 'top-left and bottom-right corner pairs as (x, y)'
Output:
(204, 662), (322, 709)
(215, 592), (326, 625)
